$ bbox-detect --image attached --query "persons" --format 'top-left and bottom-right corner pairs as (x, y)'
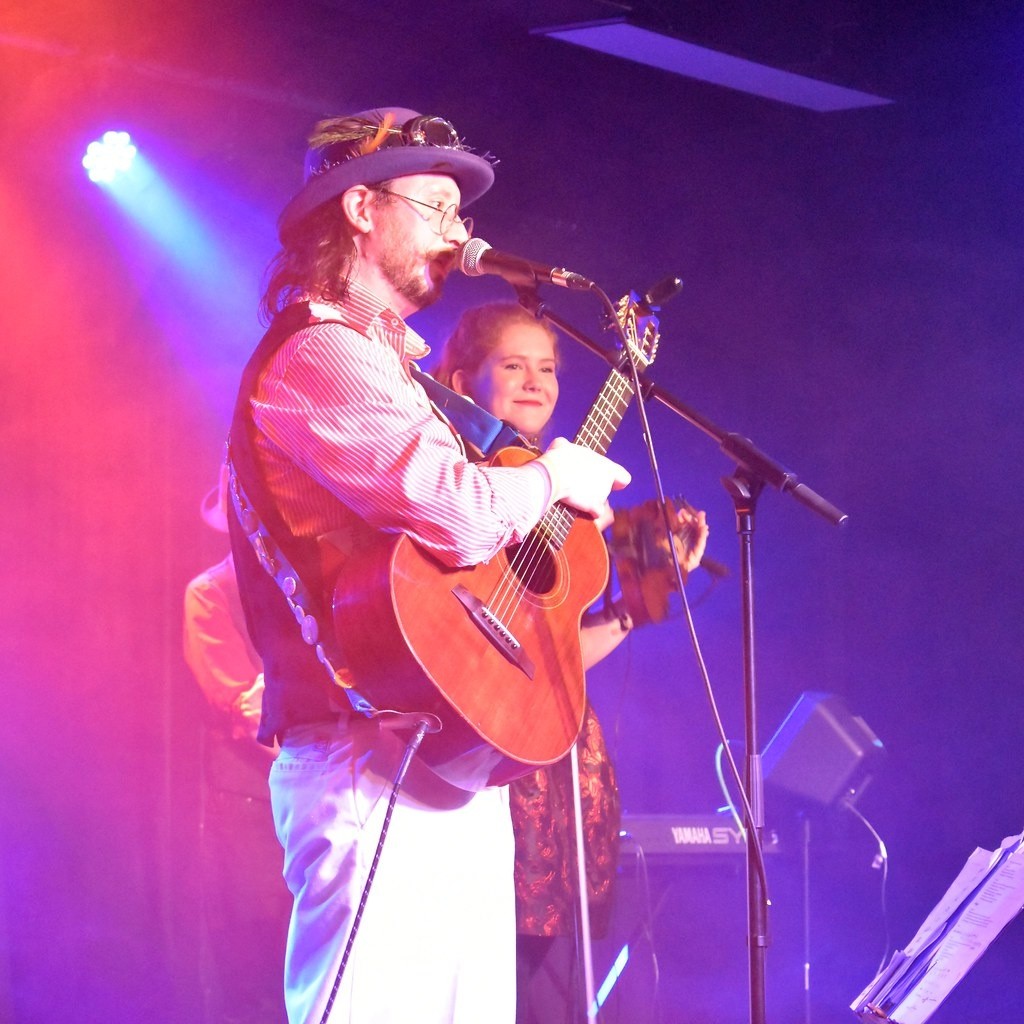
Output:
(181, 104), (710, 1024)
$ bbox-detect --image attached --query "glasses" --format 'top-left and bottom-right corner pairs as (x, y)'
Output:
(367, 184), (474, 239)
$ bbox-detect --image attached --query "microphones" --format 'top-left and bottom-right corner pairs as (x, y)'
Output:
(456, 238), (594, 291)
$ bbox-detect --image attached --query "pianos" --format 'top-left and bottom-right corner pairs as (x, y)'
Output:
(592, 809), (785, 1022)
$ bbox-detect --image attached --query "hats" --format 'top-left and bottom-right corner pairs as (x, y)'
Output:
(278, 107), (494, 250)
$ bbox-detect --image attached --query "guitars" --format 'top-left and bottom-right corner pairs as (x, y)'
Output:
(327, 295), (662, 796)
